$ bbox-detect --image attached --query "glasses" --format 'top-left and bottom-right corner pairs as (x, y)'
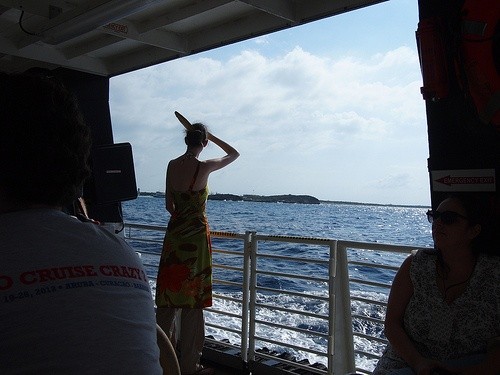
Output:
(426, 211), (468, 224)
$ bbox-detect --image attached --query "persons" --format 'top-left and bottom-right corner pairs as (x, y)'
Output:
(0, 72), (163, 375)
(371, 197), (500, 375)
(155, 123), (240, 375)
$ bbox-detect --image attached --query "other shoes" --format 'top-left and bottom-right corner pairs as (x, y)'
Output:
(197, 367), (215, 375)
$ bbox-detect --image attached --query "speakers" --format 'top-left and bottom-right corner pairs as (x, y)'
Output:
(89, 143), (138, 206)
